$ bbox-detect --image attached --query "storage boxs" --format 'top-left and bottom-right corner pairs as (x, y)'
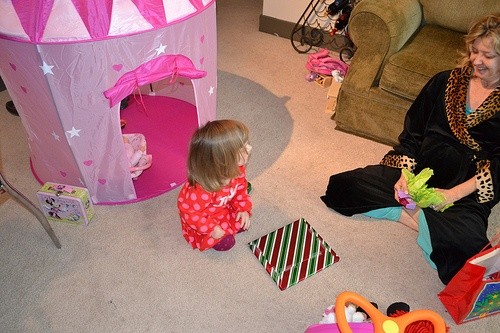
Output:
(37, 183), (95, 226)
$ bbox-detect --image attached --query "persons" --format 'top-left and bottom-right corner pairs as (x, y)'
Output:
(175, 119), (252, 253)
(320, 12), (500, 287)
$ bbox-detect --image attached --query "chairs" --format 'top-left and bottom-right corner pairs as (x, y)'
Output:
(333, 0), (500, 151)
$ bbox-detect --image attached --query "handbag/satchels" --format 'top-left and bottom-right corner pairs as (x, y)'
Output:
(437, 231), (500, 325)
